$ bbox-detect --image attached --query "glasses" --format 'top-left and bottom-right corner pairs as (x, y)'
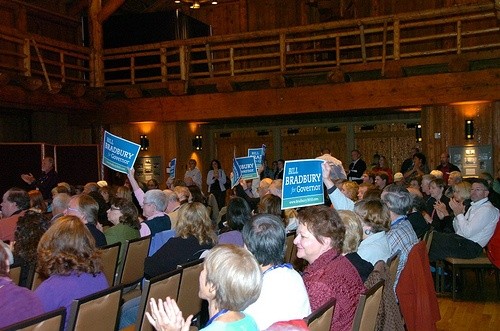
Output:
(66, 206), (81, 214)
(148, 184), (156, 187)
(111, 207), (121, 212)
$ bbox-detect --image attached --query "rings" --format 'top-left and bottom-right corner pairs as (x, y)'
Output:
(154, 319), (156, 321)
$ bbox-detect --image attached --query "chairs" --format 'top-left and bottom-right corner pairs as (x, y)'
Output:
(0, 232), (500, 331)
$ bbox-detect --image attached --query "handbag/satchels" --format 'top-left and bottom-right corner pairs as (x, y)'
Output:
(221, 169), (231, 189)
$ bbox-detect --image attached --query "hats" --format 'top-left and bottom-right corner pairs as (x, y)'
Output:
(97, 181), (108, 187)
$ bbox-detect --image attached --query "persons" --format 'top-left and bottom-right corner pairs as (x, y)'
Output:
(145, 243), (263, 331)
(241, 213), (312, 331)
(292, 204), (367, 331)
(0, 147), (500, 304)
(34, 214), (109, 331)
(118, 202), (218, 329)
(0, 244), (45, 328)
